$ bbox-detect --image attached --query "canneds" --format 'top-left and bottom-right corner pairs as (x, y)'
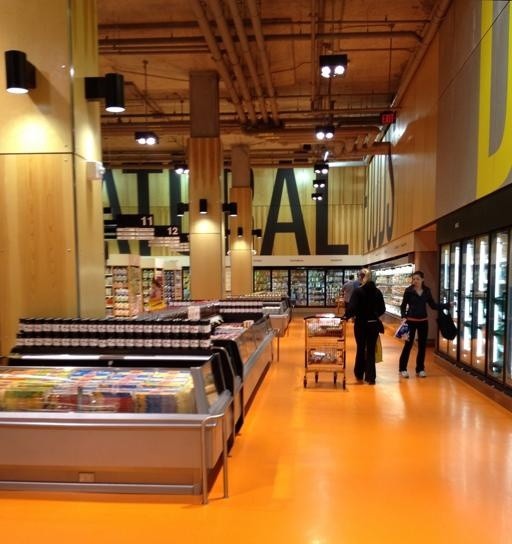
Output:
(15, 316), (213, 354)
(216, 301), (263, 317)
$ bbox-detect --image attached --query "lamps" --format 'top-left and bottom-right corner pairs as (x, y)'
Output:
(310, 1), (347, 201)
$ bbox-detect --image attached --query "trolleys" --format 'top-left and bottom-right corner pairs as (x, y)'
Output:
(336, 292), (348, 316)
(301, 312), (351, 393)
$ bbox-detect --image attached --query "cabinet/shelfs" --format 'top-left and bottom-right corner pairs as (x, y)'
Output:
(418, 182), (512, 413)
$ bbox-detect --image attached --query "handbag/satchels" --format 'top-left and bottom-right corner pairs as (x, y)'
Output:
(375, 336), (383, 363)
(436, 308), (457, 340)
(393, 320), (411, 342)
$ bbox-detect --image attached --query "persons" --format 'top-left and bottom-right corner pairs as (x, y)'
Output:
(340, 274), (356, 319)
(338, 268), (387, 386)
(398, 270), (456, 377)
(353, 271), (361, 289)
(148, 279), (162, 300)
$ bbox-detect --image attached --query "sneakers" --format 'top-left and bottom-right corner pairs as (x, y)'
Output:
(401, 371), (409, 378)
(418, 371), (427, 377)
